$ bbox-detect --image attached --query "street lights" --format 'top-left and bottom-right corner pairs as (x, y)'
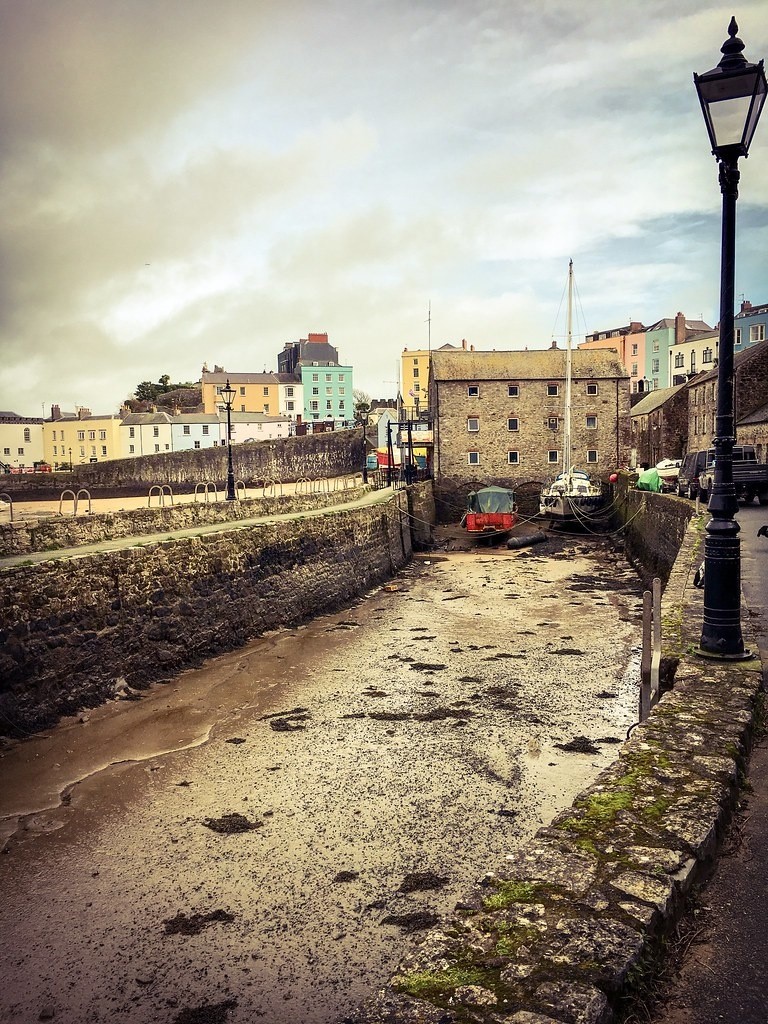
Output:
(359, 407), (369, 484)
(219, 377), (239, 500)
(685, 13), (768, 663)
(69, 447), (73, 472)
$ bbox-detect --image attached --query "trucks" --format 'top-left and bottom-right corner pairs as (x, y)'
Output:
(698, 446), (768, 505)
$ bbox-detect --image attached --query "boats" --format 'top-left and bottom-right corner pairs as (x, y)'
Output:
(463, 487), (520, 535)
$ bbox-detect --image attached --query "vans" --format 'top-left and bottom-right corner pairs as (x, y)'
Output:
(675, 450), (709, 503)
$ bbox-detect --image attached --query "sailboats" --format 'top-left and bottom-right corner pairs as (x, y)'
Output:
(539, 256), (603, 518)
